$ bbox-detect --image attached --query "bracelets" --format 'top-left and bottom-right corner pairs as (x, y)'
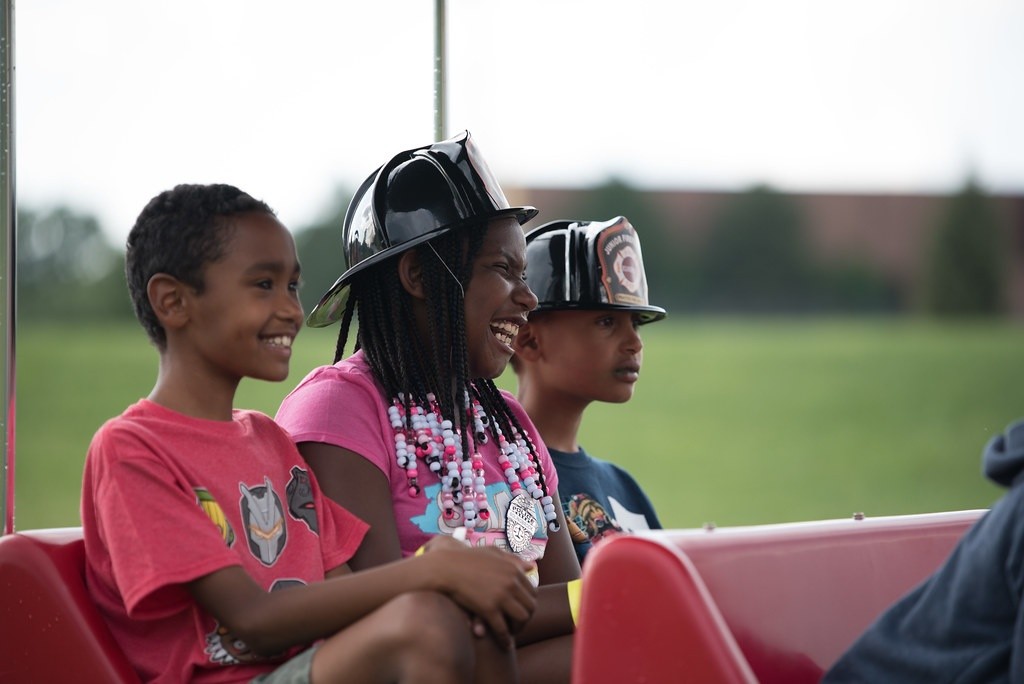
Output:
(416, 545), (424, 556)
(568, 579), (582, 627)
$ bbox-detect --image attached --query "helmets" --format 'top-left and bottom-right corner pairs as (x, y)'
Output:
(525, 216), (666, 326)
(305, 129), (540, 328)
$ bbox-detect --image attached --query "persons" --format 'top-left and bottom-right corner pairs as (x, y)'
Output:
(818, 417), (1024, 684)
(82, 129), (667, 683)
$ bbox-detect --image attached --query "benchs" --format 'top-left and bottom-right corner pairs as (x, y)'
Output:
(571, 507), (991, 684)
(0, 527), (142, 684)
